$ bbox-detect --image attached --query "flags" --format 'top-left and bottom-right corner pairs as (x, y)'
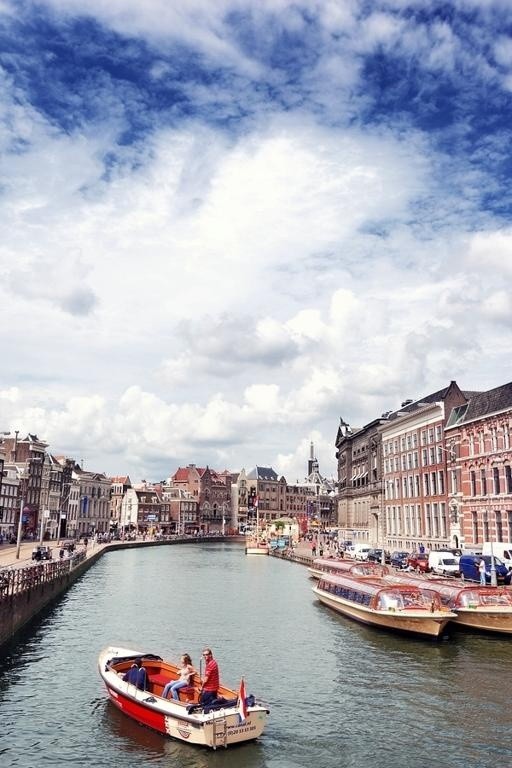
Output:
(237, 682), (248, 723)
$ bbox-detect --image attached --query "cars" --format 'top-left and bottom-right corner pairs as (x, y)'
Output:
(343, 541), (512, 585)
(31, 545), (52, 561)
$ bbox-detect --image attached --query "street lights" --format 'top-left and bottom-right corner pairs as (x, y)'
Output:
(57, 482), (74, 546)
(40, 470), (58, 545)
(222, 500), (230, 534)
(15, 457), (42, 559)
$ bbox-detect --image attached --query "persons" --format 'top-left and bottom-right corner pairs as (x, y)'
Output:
(319, 541), (324, 557)
(310, 541), (317, 556)
(122, 659), (150, 692)
(475, 557), (486, 586)
(161, 653), (196, 702)
(198, 649), (220, 703)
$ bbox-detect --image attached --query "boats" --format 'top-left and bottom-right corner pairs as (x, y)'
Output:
(98, 646), (270, 751)
(308, 557), (512, 636)
